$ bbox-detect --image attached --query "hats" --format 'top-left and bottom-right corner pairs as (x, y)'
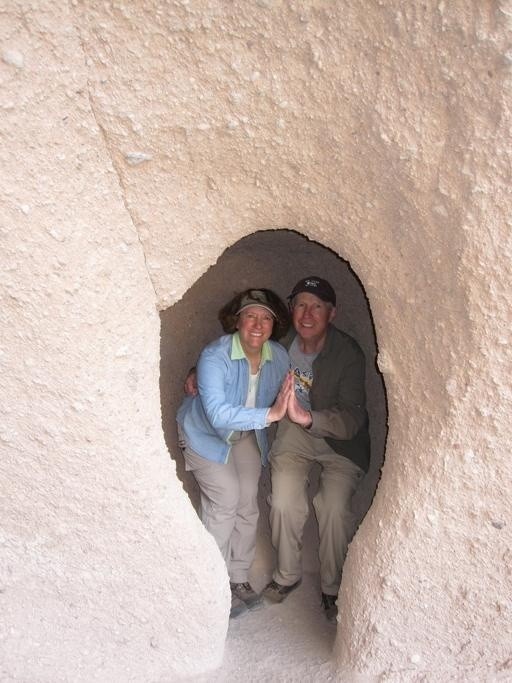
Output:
(285, 275), (338, 306)
(236, 289), (279, 318)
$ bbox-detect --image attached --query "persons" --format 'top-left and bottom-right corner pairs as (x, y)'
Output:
(175, 288), (291, 620)
(185, 276), (370, 623)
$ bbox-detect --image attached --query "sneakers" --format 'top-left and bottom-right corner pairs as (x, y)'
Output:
(261, 576), (302, 605)
(230, 589), (249, 620)
(322, 590), (340, 624)
(233, 582), (264, 612)
